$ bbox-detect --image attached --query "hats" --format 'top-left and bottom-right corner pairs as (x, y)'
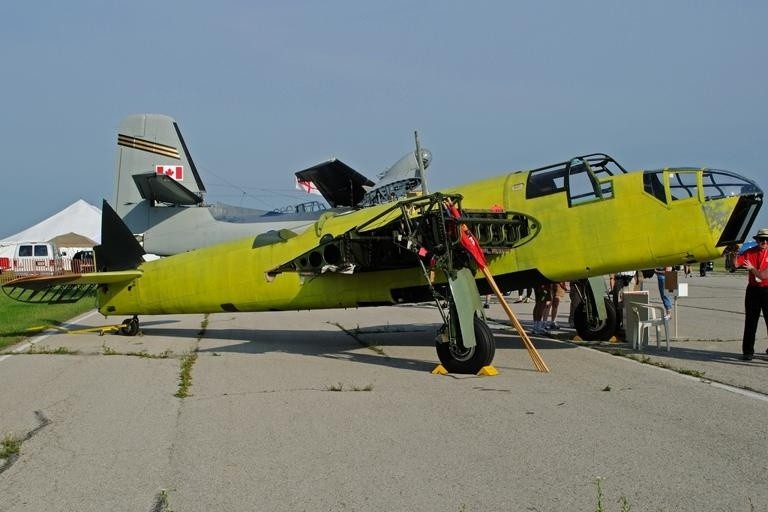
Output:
(752, 228), (768, 240)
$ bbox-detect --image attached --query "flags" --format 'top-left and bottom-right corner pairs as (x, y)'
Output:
(295, 175), (321, 196)
(154, 164), (184, 182)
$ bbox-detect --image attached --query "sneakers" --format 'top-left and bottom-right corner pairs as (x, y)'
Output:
(742, 352), (754, 360)
(532, 324), (560, 334)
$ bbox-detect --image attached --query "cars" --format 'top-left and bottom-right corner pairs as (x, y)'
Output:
(69, 249), (95, 273)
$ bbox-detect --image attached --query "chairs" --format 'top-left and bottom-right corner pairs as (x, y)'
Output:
(629, 300), (672, 352)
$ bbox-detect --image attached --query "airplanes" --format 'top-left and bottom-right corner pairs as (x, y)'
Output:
(117, 113), (432, 255)
(0, 151), (764, 373)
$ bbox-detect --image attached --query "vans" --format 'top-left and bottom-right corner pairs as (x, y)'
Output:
(15, 236), (67, 278)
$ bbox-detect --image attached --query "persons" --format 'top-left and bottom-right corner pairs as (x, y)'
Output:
(741, 258), (768, 281)
(727, 228), (768, 362)
(481, 261), (708, 337)
